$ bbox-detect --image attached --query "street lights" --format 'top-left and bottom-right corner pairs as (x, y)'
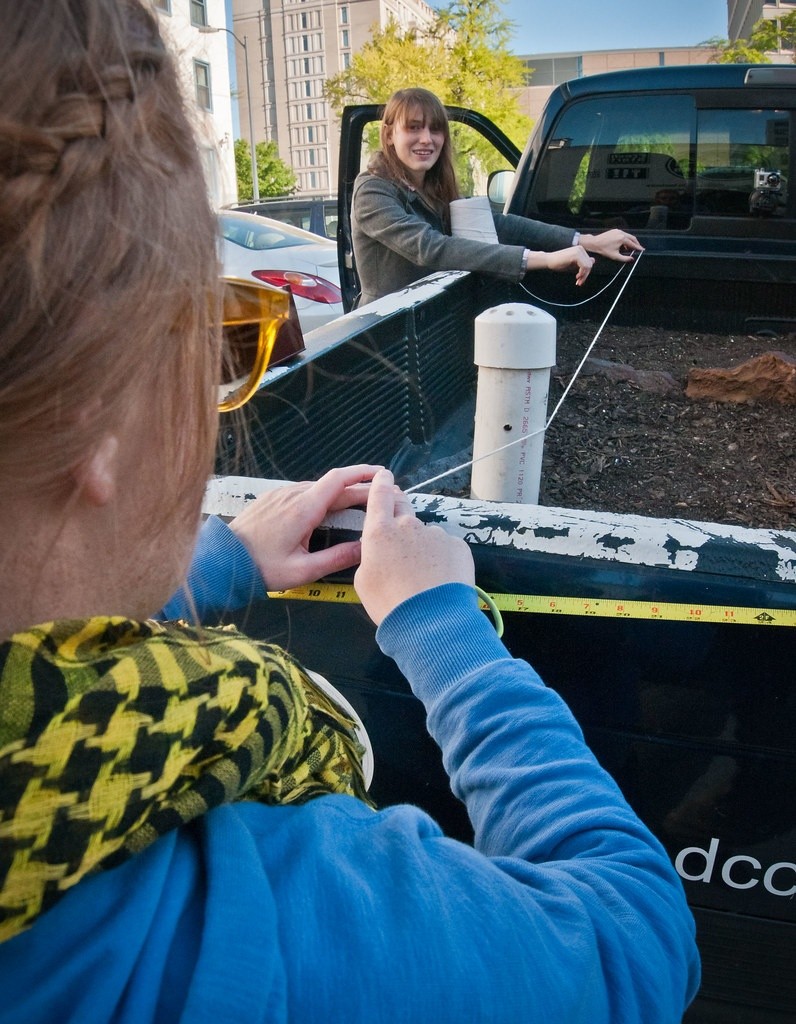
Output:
(199, 25), (261, 208)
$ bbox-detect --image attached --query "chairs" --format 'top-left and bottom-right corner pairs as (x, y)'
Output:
(582, 153), (686, 224)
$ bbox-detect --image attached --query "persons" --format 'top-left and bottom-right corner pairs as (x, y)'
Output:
(350, 88), (645, 308)
(0, 0), (702, 1024)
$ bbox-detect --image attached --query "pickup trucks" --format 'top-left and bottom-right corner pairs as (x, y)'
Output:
(200, 62), (794, 1023)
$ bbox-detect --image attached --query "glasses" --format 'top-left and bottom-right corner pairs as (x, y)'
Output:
(214, 280), (292, 417)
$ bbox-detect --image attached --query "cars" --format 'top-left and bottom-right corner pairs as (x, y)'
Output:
(215, 212), (345, 336)
(228, 195), (337, 246)
